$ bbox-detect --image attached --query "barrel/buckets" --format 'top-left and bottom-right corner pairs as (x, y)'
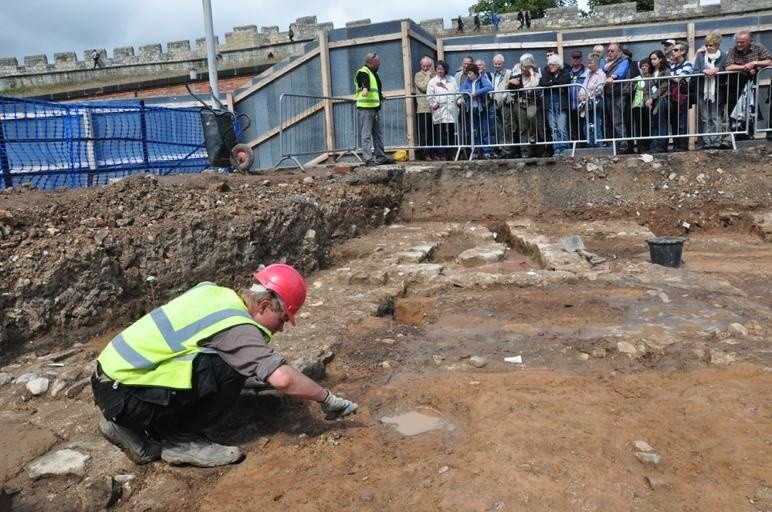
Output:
(644, 235), (690, 269)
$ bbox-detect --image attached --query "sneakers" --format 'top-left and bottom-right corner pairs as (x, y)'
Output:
(161, 436), (242, 467)
(98, 417), (161, 464)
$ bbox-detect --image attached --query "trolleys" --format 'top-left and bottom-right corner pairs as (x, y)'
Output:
(184, 82), (254, 175)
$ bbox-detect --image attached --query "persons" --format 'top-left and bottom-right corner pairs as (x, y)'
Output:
(414, 31), (771, 164)
(287, 25), (294, 42)
(90, 260), (358, 468)
(456, 8), (532, 34)
(90, 48), (101, 67)
(352, 52), (397, 165)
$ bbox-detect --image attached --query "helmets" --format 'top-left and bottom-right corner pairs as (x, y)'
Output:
(253, 264), (306, 327)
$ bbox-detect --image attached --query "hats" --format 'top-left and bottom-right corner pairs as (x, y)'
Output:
(662, 40), (675, 46)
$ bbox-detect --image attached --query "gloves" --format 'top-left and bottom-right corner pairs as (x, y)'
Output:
(317, 389), (356, 420)
